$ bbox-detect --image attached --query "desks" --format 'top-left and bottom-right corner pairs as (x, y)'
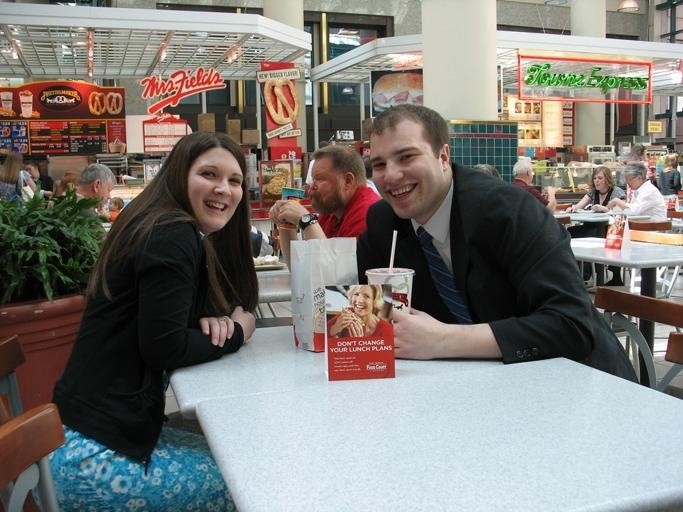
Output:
(128, 165), (144, 178)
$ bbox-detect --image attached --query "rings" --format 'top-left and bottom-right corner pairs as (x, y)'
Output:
(218, 317), (229, 322)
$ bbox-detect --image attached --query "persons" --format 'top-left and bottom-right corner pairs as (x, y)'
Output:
(306, 140), (327, 185)
(563, 165), (625, 282)
(657, 151), (681, 213)
(31, 131), (260, 511)
(472, 160), (500, 181)
(591, 163), (668, 287)
(0, 151), (124, 223)
(250, 209), (275, 259)
(508, 157), (557, 215)
(357, 105), (640, 379)
(327, 285), (391, 337)
(623, 142), (653, 201)
(365, 159), (380, 198)
(268, 144), (378, 276)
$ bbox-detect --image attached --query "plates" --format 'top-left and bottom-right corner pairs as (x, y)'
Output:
(253, 260), (287, 271)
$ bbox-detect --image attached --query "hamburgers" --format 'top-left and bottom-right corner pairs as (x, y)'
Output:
(373, 72), (423, 112)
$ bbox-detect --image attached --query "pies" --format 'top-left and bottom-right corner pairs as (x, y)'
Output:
(341, 307), (365, 329)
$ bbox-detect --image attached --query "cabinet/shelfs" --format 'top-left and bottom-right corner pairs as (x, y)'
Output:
(97, 157), (128, 177)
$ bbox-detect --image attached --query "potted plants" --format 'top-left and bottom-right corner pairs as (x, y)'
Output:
(0, 180), (106, 425)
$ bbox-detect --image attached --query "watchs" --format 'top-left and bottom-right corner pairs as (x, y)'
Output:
(296, 214), (319, 233)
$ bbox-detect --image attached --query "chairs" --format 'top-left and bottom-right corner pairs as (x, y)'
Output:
(0, 336), (26, 425)
(554, 198), (683, 397)
(268, 236), (280, 256)
(0, 403), (66, 512)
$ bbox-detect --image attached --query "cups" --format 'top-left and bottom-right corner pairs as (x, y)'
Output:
(109, 142), (126, 153)
(279, 185), (306, 231)
(365, 267), (415, 324)
(0, 93), (12, 112)
(19, 95), (33, 118)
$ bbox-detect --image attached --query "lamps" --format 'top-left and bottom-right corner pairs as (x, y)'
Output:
(617, 0), (640, 12)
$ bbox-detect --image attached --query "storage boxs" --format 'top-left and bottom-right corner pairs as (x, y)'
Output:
(242, 130), (260, 144)
(198, 113), (215, 132)
(227, 120), (240, 143)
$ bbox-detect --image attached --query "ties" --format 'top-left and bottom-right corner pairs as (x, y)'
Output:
(410, 225), (477, 327)
